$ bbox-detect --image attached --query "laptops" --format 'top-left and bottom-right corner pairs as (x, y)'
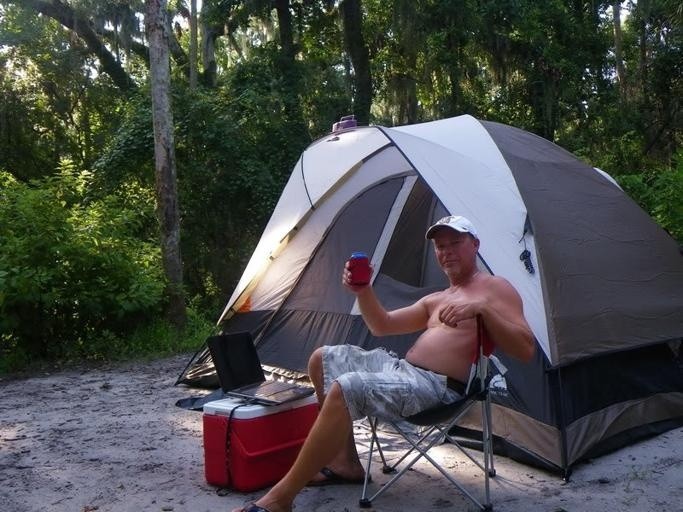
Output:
(208, 331), (315, 406)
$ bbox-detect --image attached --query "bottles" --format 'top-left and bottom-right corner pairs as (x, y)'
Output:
(349, 250), (369, 285)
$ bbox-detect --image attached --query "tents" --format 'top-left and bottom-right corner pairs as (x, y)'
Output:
(172, 112), (681, 485)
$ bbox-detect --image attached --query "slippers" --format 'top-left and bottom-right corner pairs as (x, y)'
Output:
(305, 466), (372, 488)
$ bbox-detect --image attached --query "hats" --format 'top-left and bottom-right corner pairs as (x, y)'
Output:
(424, 214), (478, 243)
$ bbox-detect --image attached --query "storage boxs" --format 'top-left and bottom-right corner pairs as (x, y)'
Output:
(203, 394), (320, 492)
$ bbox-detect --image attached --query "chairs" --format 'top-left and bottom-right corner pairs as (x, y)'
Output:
(359, 313), (496, 512)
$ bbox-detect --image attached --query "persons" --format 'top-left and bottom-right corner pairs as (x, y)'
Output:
(230, 215), (536, 512)
